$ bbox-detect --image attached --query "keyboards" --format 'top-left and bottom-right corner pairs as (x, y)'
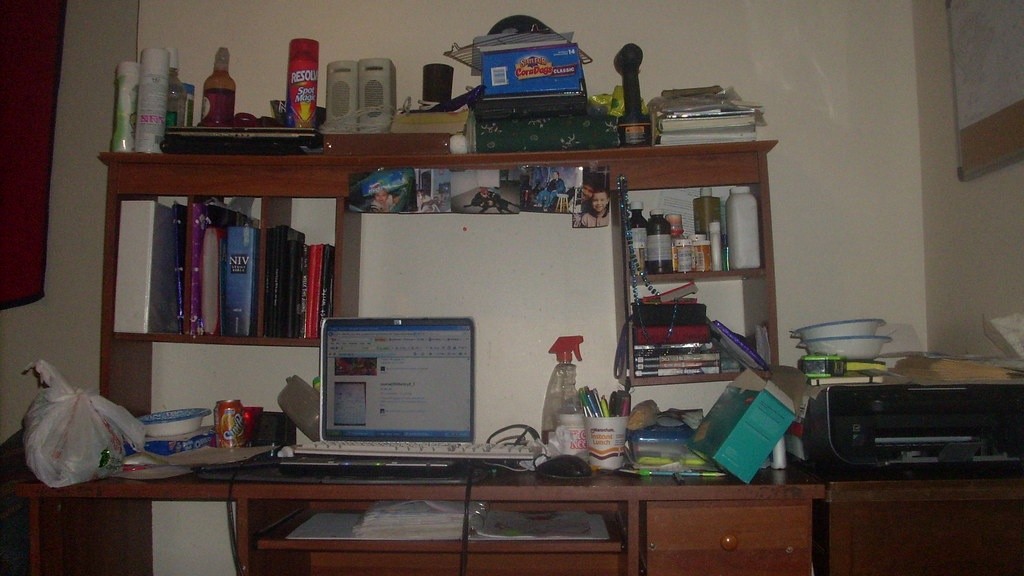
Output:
(295, 440), (542, 460)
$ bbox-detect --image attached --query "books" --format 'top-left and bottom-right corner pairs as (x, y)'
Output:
(895, 355), (1023, 385)
(391, 104), (468, 133)
(352, 501), (591, 540)
(846, 360), (886, 370)
(808, 369), (884, 387)
(114, 200), (335, 340)
(656, 85), (757, 145)
(633, 283), (769, 378)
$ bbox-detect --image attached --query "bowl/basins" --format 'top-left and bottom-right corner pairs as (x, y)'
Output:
(134, 407), (212, 438)
(279, 375), (320, 442)
(790, 318), (886, 341)
(796, 336), (893, 359)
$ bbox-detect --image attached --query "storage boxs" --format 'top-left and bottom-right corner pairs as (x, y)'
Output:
(481, 40), (584, 97)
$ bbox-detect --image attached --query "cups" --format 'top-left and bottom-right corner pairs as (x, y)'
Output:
(556, 413), (588, 461)
(423, 63), (454, 104)
(585, 416), (631, 471)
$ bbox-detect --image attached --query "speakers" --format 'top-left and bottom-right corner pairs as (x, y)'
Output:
(321, 61), (360, 134)
(358, 58), (396, 134)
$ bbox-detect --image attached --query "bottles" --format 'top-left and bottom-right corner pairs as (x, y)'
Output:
(645, 187), (729, 274)
(285, 38), (320, 128)
(628, 201), (648, 276)
(770, 435), (787, 470)
(201, 46), (236, 126)
(111, 45), (196, 154)
(725, 186), (761, 271)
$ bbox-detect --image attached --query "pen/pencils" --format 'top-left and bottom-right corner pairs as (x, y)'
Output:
(577, 386), (617, 417)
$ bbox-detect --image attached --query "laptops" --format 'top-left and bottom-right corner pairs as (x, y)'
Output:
(280, 316), (476, 467)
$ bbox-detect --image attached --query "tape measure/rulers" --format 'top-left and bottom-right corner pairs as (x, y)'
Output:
(797, 353), (847, 379)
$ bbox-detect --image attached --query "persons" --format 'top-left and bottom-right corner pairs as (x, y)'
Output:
(573, 177), (609, 228)
(533, 171), (564, 213)
(416, 189), (425, 211)
(472, 187), (519, 213)
(369, 187), (400, 213)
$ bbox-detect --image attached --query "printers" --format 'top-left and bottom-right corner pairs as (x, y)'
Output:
(801, 381), (1024, 482)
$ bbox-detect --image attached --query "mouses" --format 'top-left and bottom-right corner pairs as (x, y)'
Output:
(537, 454), (592, 478)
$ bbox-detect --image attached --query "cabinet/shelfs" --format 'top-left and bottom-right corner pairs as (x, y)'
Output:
(96, 137), (780, 393)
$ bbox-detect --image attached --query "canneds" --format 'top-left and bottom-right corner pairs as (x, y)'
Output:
(213, 398), (245, 448)
(674, 239), (711, 273)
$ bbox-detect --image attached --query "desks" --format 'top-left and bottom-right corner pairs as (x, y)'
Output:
(14, 442), (1024, 576)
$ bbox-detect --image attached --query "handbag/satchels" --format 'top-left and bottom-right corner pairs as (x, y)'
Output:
(22, 359), (145, 489)
